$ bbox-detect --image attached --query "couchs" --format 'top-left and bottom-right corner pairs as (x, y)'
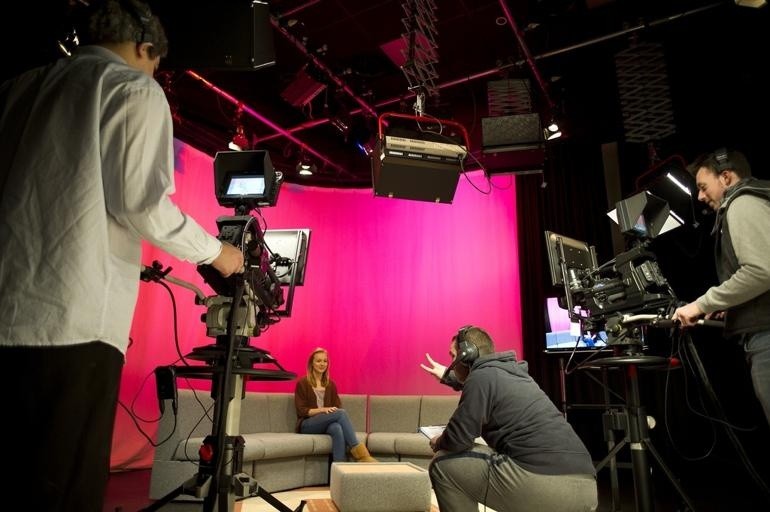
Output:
(148, 389), (461, 501)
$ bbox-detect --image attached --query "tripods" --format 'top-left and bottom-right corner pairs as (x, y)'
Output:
(596, 365), (697, 512)
(133, 378), (306, 512)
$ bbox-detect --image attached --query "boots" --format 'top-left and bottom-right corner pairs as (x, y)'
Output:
(350, 442), (380, 463)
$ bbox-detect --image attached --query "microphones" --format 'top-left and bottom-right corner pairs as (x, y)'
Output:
(439, 351), (467, 383)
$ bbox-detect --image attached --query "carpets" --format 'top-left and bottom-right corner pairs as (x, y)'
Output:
(235, 487), (441, 512)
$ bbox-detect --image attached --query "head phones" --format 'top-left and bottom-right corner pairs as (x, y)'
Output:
(458, 326), (479, 362)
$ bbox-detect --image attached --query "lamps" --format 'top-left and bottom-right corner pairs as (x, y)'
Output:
(297, 153), (317, 176)
(542, 117), (563, 142)
(57, 28), (81, 56)
(228, 108), (249, 151)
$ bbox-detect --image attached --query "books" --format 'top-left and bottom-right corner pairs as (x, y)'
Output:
(419, 425), (447, 440)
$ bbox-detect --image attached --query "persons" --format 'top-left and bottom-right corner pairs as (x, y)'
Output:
(419, 325), (599, 512)
(294, 346), (379, 463)
(672, 145), (770, 436)
(2, 2), (248, 512)
